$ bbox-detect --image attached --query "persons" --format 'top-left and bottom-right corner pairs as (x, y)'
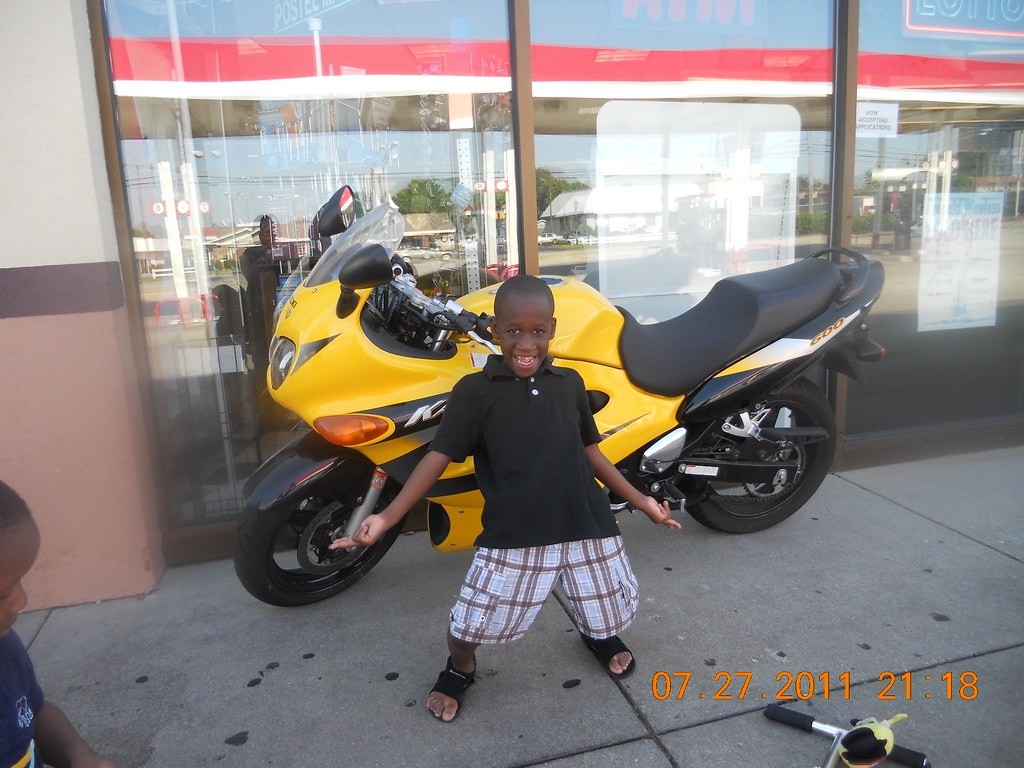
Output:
(0, 479), (120, 768)
(894, 199), (912, 256)
(240, 216), (299, 441)
(329, 274), (682, 723)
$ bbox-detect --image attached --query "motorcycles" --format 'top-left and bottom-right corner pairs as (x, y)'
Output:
(227, 187), (886, 607)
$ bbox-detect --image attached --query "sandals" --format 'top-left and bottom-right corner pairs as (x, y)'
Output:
(427, 654), (477, 722)
(578, 630), (635, 678)
